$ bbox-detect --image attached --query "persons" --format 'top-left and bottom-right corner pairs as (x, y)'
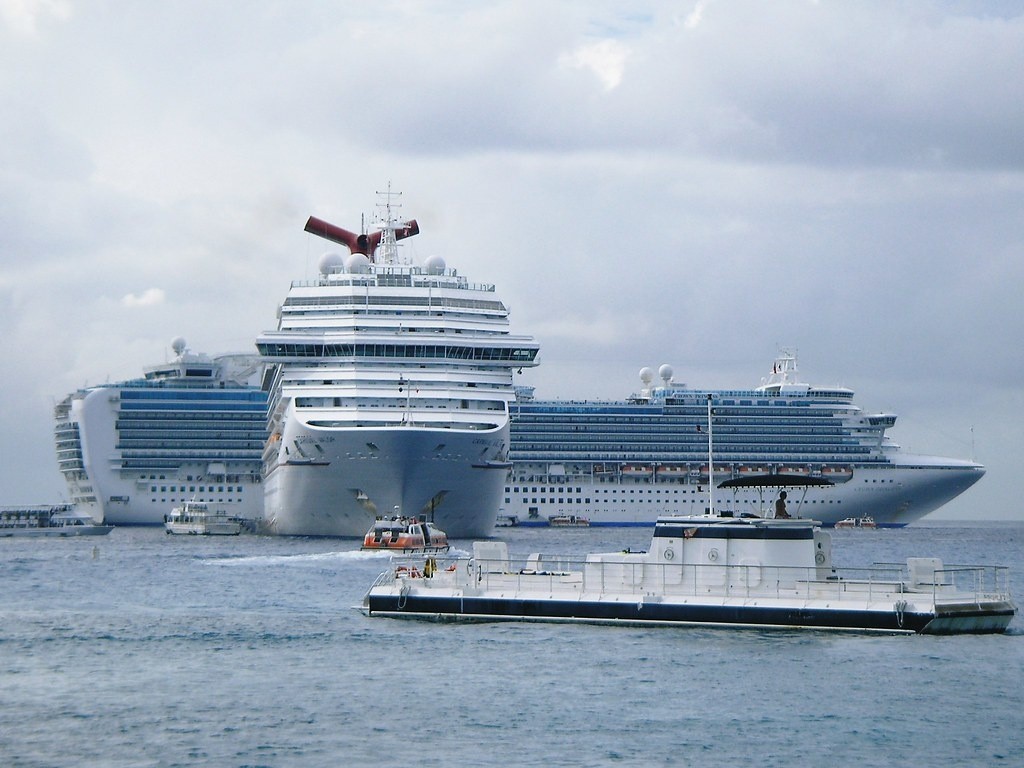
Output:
(775, 491), (790, 519)
(31, 506), (107, 527)
(164, 513), (194, 524)
(376, 513), (418, 533)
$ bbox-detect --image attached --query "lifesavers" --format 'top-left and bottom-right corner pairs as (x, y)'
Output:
(394, 565), (410, 579)
(423, 558), (435, 577)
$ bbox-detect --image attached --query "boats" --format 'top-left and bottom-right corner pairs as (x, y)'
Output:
(163, 493), (241, 536)
(0, 501), (117, 538)
(351, 390), (1021, 637)
(359, 513), (450, 550)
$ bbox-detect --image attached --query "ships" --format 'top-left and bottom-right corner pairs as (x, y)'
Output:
(53, 179), (990, 540)
